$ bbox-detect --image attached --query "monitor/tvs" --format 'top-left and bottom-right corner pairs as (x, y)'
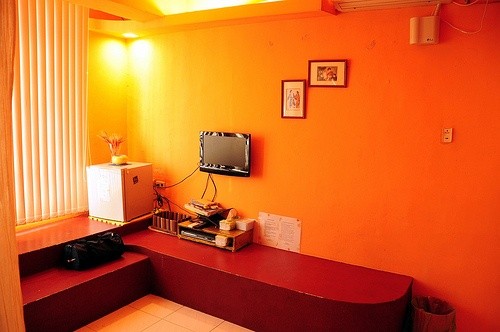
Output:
(199, 131), (251, 177)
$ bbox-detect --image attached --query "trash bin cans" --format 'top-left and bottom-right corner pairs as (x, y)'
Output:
(410, 295), (455, 332)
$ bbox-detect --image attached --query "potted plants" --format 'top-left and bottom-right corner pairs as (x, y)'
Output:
(98, 130), (129, 165)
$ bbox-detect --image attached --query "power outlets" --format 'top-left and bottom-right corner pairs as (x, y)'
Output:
(156, 181), (166, 188)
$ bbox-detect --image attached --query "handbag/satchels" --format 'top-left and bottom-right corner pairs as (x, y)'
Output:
(64, 231), (124, 269)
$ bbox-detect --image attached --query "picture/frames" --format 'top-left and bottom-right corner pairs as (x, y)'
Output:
(281, 79), (306, 119)
(307, 59), (347, 88)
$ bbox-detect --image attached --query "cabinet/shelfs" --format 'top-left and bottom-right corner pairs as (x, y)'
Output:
(85, 161), (154, 222)
(177, 218), (253, 252)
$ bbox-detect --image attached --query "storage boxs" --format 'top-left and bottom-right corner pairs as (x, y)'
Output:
(153, 210), (191, 236)
